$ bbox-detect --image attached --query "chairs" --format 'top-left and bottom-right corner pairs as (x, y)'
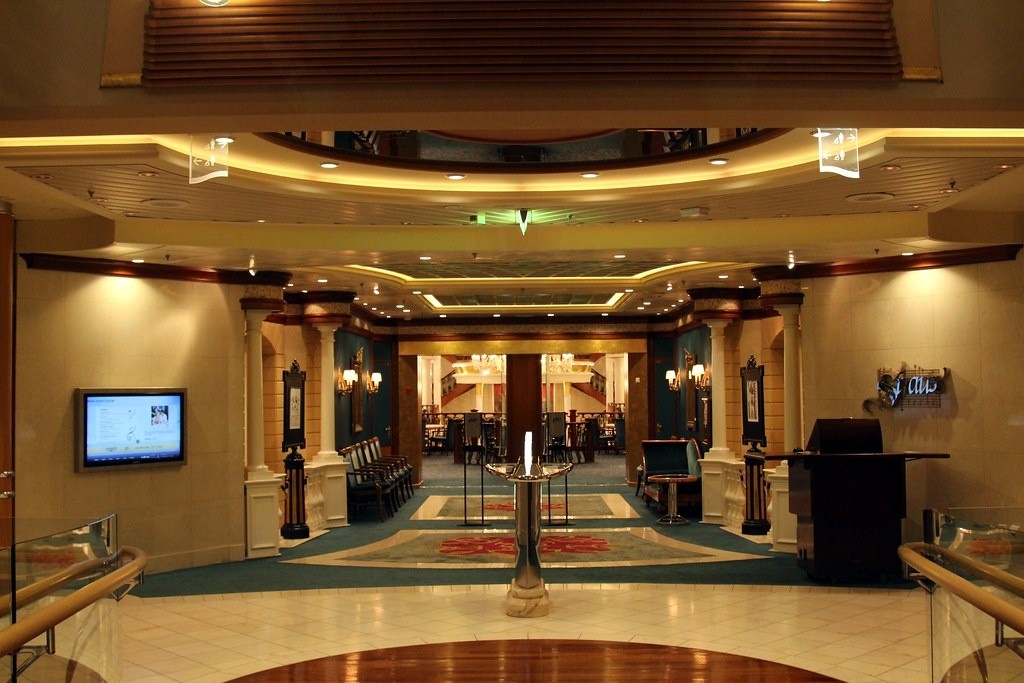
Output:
(636, 439), (703, 515)
(421, 412), (626, 465)
(337, 436), (414, 522)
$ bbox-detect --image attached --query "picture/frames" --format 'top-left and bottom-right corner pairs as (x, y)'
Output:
(740, 364), (766, 445)
(282, 359), (307, 452)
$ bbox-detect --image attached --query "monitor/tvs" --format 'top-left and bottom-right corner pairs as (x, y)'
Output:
(74, 388), (189, 473)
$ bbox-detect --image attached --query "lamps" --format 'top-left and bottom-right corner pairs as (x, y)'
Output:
(692, 364), (709, 391)
(249, 269), (257, 275)
(786, 262), (795, 268)
(665, 370), (682, 391)
(367, 370), (382, 393)
(337, 370), (359, 395)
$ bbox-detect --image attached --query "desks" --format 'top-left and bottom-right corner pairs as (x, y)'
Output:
(647, 474), (696, 527)
